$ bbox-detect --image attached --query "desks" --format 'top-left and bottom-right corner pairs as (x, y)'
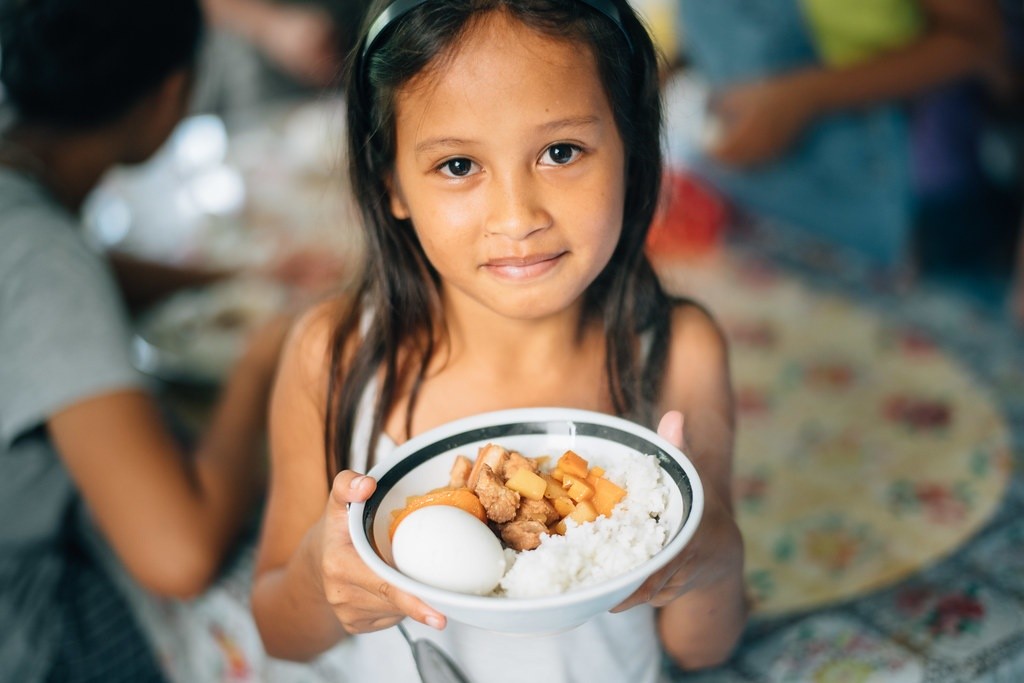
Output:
(57, 226), (1024, 683)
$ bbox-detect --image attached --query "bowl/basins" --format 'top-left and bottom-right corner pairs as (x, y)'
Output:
(348, 407), (703, 636)
(130, 279), (288, 401)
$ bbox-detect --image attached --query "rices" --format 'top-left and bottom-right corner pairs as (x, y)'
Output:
(487, 450), (670, 600)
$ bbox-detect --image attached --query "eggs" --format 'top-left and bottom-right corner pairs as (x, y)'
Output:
(391, 504), (508, 600)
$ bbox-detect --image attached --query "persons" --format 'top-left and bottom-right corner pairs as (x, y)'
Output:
(249, 0), (748, 683)
(199, 0), (366, 107)
(677, 0), (999, 335)
(0, 0), (289, 683)
